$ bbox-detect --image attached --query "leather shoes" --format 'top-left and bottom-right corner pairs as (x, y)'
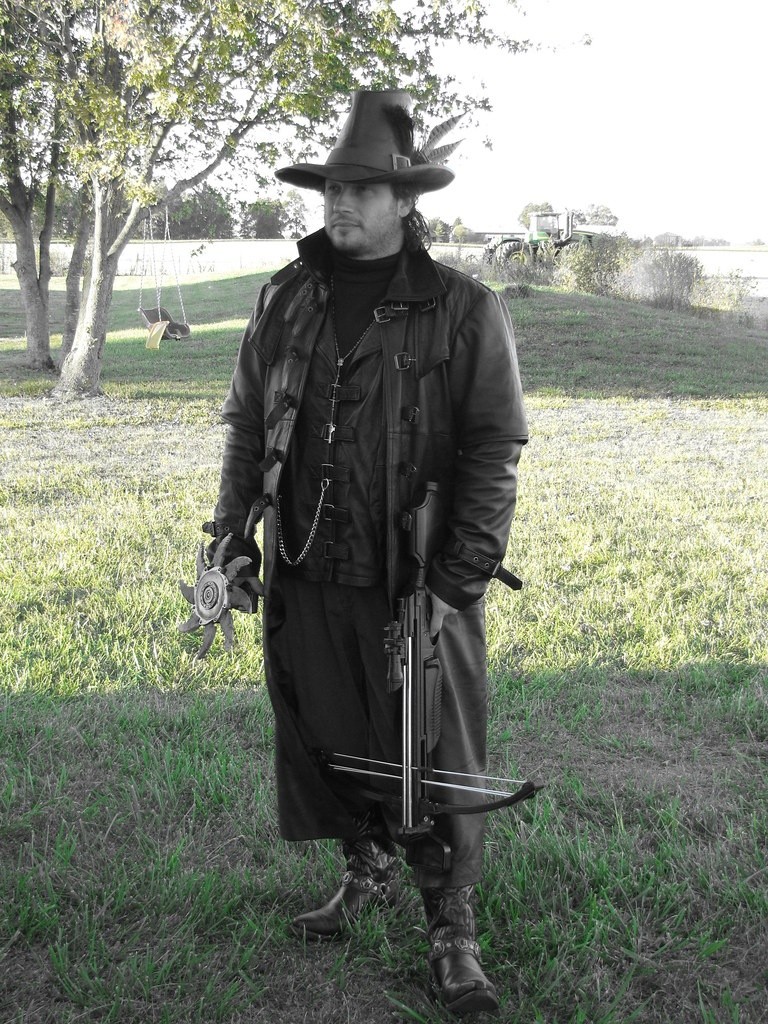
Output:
(289, 870), (389, 941)
(428, 938), (499, 1011)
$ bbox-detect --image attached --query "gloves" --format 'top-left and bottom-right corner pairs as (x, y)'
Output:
(207, 535), (264, 595)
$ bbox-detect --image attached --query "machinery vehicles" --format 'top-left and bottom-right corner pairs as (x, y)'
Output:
(482, 211), (594, 270)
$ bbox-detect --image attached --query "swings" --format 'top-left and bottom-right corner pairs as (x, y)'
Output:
(138, 206), (192, 343)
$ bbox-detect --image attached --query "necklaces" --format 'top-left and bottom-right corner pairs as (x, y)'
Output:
(328, 274), (378, 441)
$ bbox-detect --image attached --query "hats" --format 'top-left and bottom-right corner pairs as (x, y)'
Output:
(276, 89), (453, 189)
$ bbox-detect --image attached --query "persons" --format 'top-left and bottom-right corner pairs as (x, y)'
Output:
(202, 89), (530, 1011)
